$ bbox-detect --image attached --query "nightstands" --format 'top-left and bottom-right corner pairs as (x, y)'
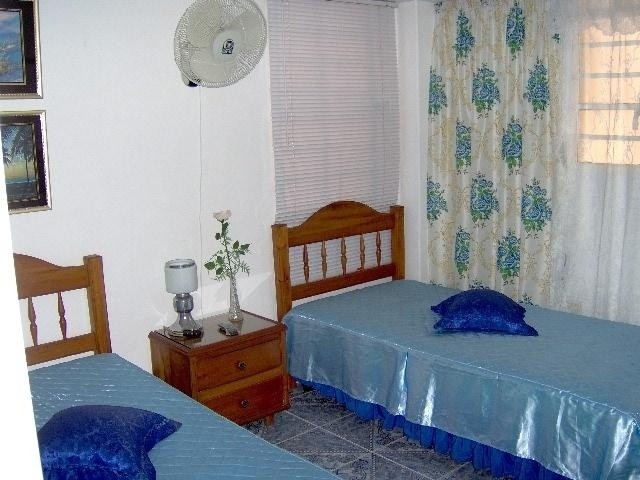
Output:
(143, 303), (295, 429)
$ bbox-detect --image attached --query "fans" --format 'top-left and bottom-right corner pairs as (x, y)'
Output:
(174, 0), (269, 90)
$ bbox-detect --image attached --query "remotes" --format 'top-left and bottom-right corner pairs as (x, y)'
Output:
(217, 320), (240, 336)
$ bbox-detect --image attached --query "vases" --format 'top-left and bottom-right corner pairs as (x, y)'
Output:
(225, 274), (246, 324)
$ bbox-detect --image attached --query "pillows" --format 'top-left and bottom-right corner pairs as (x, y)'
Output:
(36, 402), (183, 480)
(426, 283), (541, 338)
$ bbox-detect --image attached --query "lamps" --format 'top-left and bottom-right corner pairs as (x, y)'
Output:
(159, 256), (205, 339)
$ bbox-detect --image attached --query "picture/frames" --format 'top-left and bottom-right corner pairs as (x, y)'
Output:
(0, 109), (53, 216)
(0, 0), (44, 99)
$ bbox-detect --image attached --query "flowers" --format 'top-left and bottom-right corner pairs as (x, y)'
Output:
(201, 207), (252, 282)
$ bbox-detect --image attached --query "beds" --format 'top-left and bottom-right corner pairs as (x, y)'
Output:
(269, 196), (640, 480)
(12, 245), (345, 480)
